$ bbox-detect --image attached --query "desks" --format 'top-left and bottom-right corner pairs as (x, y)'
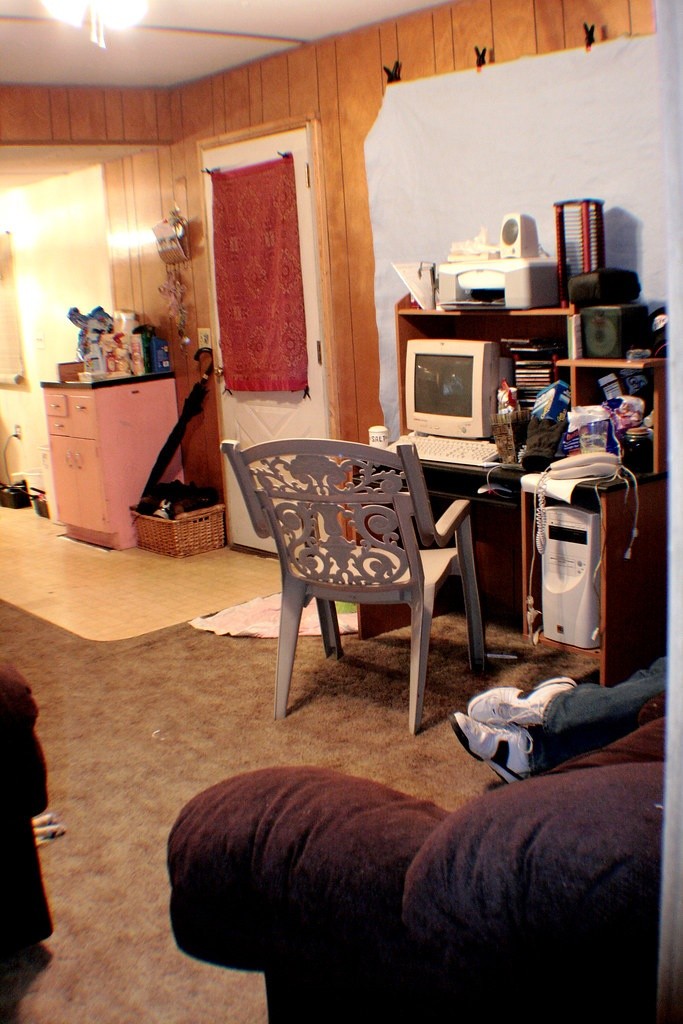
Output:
(347, 292), (668, 687)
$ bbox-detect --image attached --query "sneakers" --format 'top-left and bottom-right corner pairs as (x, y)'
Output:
(468, 675), (578, 727)
(451, 712), (535, 787)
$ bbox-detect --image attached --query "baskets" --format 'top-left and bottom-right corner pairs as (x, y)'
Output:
(129, 499), (226, 561)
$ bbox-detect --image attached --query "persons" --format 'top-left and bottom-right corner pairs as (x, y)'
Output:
(448, 655), (667, 784)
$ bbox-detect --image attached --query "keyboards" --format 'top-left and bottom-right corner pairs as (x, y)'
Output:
(385, 437), (500, 465)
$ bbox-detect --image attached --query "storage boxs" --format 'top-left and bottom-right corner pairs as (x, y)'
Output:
(0, 487), (30, 509)
(531, 380), (572, 423)
(597, 368), (652, 401)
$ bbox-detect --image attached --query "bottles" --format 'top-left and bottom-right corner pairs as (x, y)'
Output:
(623, 428), (653, 473)
(106, 352), (115, 372)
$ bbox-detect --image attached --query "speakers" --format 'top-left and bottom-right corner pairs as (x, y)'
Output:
(582, 302), (650, 358)
(500, 213), (537, 257)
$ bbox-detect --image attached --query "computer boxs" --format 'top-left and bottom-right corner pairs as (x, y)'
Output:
(541, 501), (600, 649)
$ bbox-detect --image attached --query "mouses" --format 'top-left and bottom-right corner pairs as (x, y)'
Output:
(477, 482), (514, 497)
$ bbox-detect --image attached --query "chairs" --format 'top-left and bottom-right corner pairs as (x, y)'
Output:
(218, 437), (486, 735)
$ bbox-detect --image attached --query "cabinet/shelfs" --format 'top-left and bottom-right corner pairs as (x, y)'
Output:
(40, 371), (185, 551)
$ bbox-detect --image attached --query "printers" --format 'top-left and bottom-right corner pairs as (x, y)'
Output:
(438, 258), (558, 310)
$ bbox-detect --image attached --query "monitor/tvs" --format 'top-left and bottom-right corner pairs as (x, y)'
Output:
(405, 338), (513, 440)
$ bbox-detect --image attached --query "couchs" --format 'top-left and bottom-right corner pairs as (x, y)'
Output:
(168, 692), (666, 1023)
(1, 660), (53, 962)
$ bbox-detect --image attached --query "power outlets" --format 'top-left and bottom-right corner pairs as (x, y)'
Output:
(14, 426), (21, 440)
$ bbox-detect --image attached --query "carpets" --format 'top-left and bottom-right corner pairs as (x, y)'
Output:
(1, 589), (601, 1024)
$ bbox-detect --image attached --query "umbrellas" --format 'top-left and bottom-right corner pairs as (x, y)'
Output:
(136, 347), (214, 515)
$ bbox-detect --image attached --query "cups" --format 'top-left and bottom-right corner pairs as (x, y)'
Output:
(369, 426), (388, 450)
(578, 421), (608, 454)
